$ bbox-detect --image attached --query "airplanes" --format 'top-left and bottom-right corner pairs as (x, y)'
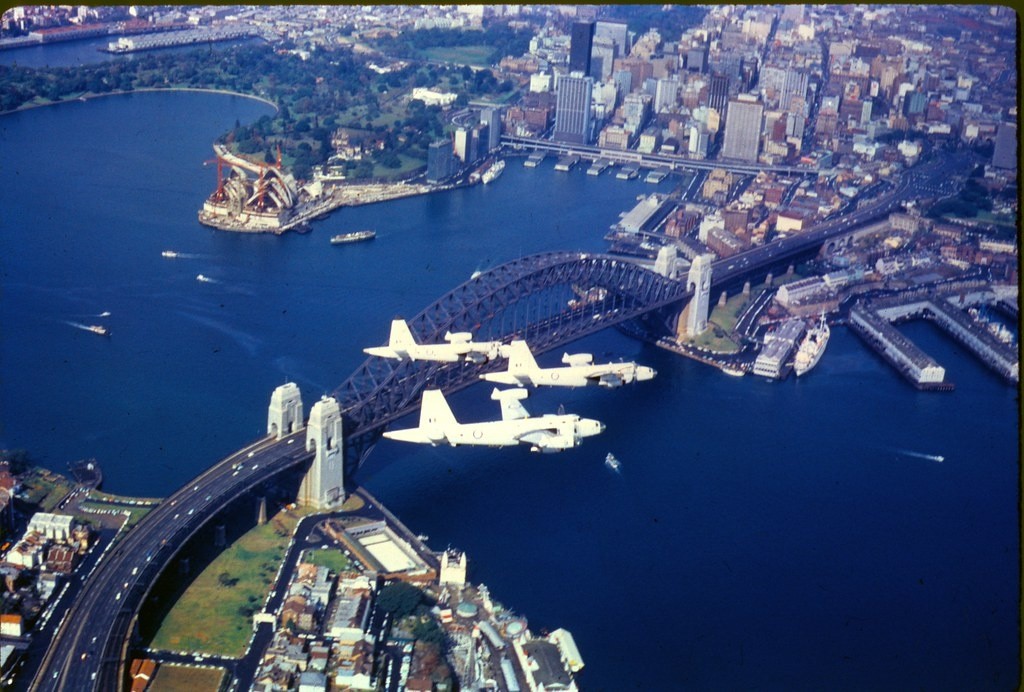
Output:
(362, 319), (511, 364)
(382, 386), (607, 452)
(478, 341), (658, 387)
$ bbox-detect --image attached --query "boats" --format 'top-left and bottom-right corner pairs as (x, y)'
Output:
(331, 229), (377, 244)
(481, 160), (505, 185)
(102, 311), (109, 316)
(197, 274), (206, 281)
(792, 315), (830, 377)
(160, 250), (177, 258)
(89, 326), (107, 334)
